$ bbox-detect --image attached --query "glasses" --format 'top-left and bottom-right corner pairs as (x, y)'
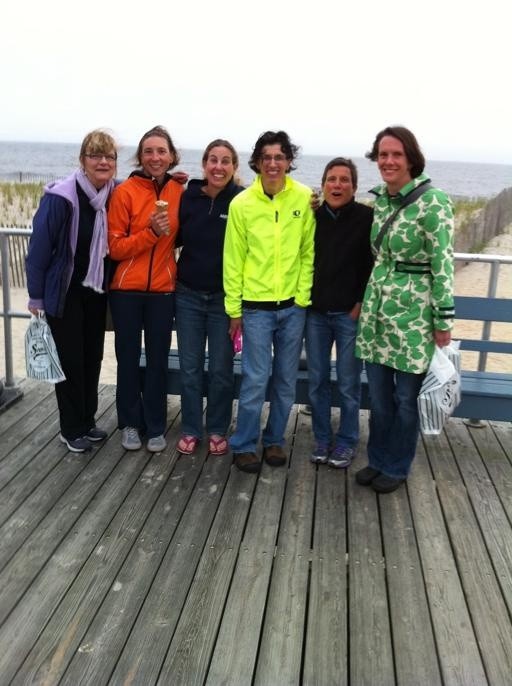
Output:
(84, 152), (116, 161)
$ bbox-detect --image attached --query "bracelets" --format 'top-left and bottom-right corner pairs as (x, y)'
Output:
(150, 225), (160, 238)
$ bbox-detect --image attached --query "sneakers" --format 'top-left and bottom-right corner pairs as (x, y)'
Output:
(309, 443), (407, 494)
(234, 451), (261, 473)
(59, 431), (92, 453)
(263, 445), (286, 466)
(83, 428), (108, 442)
(122, 426), (142, 451)
(146, 435), (168, 452)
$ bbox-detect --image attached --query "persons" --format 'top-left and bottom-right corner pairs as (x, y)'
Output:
(354, 127), (457, 496)
(26, 132), (190, 453)
(171, 139), (319, 458)
(307, 157), (369, 469)
(222, 131), (316, 473)
(105, 124), (183, 453)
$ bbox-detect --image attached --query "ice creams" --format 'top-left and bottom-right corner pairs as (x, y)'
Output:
(154, 200), (168, 215)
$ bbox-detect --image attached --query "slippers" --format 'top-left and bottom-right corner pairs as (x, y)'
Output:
(208, 433), (228, 455)
(176, 434), (198, 455)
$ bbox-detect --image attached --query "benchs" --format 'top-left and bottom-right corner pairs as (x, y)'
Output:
(129, 293), (512, 424)
